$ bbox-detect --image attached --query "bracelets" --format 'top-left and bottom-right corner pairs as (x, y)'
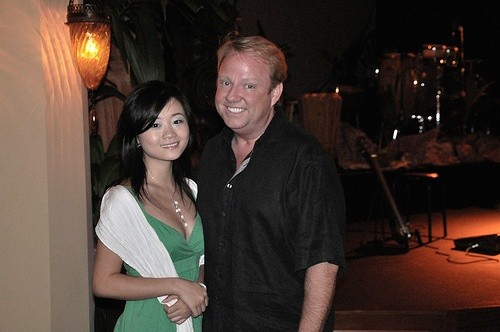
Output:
(198, 282), (207, 289)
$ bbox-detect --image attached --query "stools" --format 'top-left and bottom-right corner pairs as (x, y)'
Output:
(374, 171), (447, 247)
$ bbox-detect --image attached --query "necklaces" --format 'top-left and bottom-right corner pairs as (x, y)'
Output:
(147, 170), (187, 228)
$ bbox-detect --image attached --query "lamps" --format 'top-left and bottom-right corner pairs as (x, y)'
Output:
(64, 0), (112, 136)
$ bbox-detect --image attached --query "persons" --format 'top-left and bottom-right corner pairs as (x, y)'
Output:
(193, 35), (346, 332)
(92, 80), (208, 332)
